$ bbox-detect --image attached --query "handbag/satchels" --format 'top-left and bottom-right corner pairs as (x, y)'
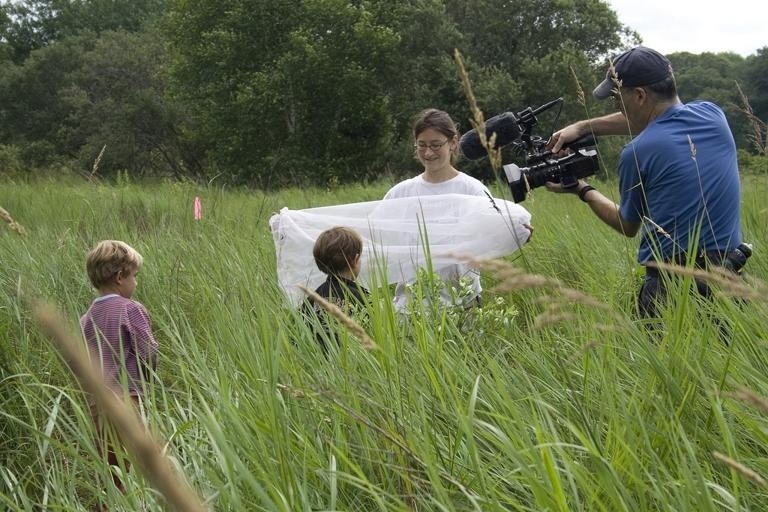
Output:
(578, 185), (596, 203)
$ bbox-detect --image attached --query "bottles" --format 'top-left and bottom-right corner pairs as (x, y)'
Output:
(592, 46), (672, 100)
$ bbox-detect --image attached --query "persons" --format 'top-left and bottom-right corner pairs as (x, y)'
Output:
(269, 109), (535, 359)
(285, 227), (374, 366)
(79, 240), (161, 498)
(540, 44), (753, 353)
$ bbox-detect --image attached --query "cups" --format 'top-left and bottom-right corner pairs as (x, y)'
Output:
(413, 138), (450, 152)
